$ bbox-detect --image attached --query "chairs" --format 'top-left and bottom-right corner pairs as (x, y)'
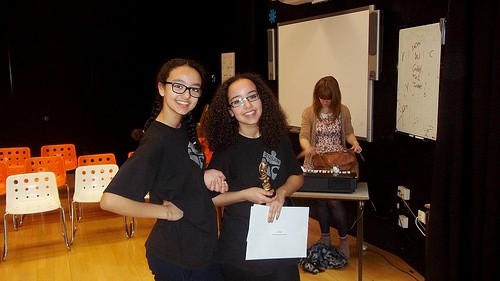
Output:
(77, 153), (117, 223)
(70, 164), (134, 245)
(41, 144), (78, 211)
(0, 147), (31, 183)
(0, 162), (18, 231)
(19, 156), (72, 227)
(1, 171), (71, 261)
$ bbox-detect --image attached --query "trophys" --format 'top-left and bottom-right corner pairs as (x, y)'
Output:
(259, 163), (276, 199)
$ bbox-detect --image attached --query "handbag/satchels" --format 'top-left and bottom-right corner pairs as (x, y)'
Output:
(312, 150), (357, 171)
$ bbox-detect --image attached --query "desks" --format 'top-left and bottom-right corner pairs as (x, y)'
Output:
(290, 182), (370, 281)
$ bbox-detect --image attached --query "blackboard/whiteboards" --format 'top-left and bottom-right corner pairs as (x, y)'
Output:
(394, 22), (443, 140)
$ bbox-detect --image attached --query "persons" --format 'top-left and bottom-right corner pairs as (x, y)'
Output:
(300, 76), (362, 260)
(99, 59), (228, 281)
(203, 73), (305, 281)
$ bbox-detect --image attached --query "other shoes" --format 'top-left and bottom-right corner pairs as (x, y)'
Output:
(315, 235), (331, 247)
(339, 235), (348, 257)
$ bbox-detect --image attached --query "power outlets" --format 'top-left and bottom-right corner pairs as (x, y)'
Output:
(398, 215), (408, 228)
(418, 210), (426, 225)
(397, 186), (410, 201)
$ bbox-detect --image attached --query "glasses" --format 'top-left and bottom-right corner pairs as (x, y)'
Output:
(228, 94), (260, 108)
(164, 81), (203, 98)
